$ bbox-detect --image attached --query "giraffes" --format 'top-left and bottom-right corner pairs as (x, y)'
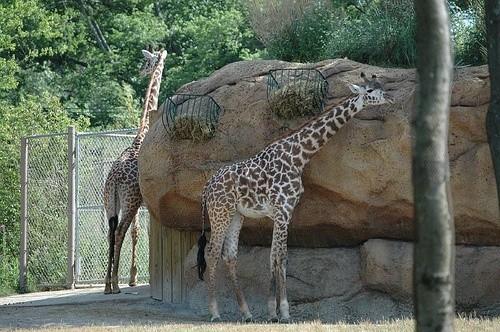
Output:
(103, 43), (170, 295)
(195, 70), (397, 325)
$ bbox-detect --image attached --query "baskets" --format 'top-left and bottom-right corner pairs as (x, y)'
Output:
(267, 68), (329, 118)
(162, 94), (220, 141)
